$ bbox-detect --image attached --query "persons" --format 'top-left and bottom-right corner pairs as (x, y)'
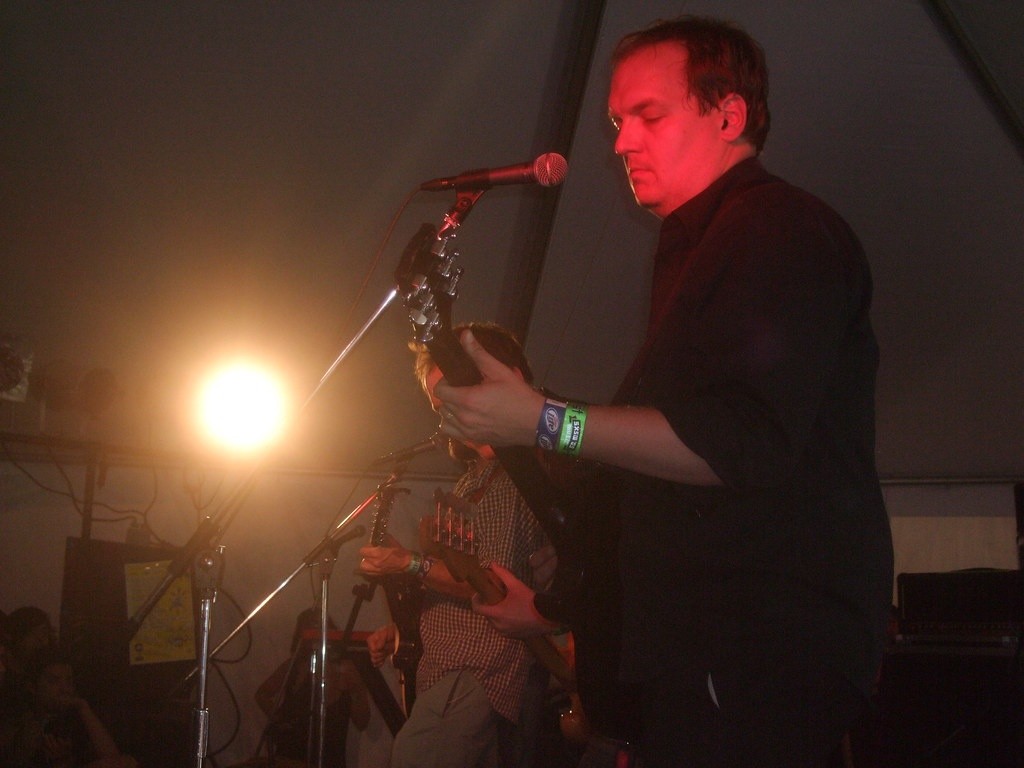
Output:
(359, 15), (896, 767)
(0, 605), (140, 768)
(254, 605), (372, 767)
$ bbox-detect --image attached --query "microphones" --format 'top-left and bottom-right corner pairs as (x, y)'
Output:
(377, 432), (447, 467)
(421, 153), (569, 193)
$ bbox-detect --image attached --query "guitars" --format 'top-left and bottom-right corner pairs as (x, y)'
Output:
(399, 221), (594, 632)
(370, 484), (422, 717)
(426, 495), (630, 756)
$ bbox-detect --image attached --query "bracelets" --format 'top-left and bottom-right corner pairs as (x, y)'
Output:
(533, 397), (590, 460)
(405, 550), (435, 582)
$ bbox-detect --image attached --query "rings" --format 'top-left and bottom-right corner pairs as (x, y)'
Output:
(444, 412), (456, 423)
